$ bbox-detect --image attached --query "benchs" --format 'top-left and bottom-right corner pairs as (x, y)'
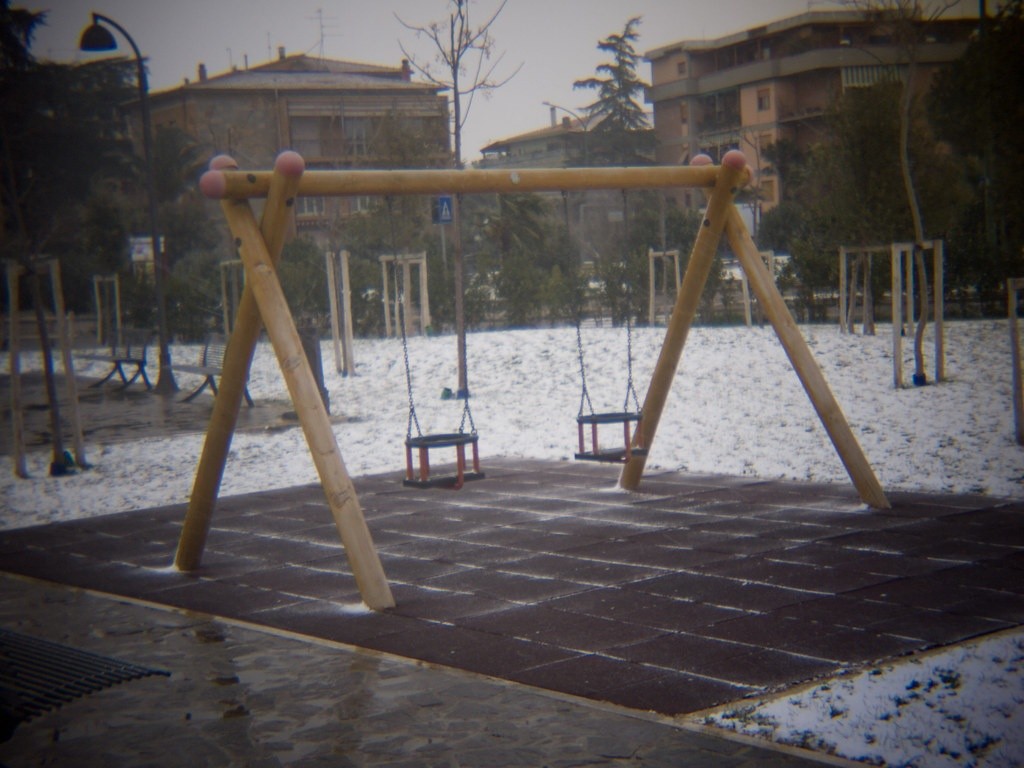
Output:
(164, 332), (258, 402)
(78, 328), (156, 393)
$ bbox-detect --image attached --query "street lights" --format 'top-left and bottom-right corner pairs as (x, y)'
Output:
(543, 102), (590, 167)
(79, 10), (175, 389)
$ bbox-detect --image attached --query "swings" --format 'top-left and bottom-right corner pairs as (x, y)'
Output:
(384, 197), (486, 491)
(563, 195), (649, 463)
